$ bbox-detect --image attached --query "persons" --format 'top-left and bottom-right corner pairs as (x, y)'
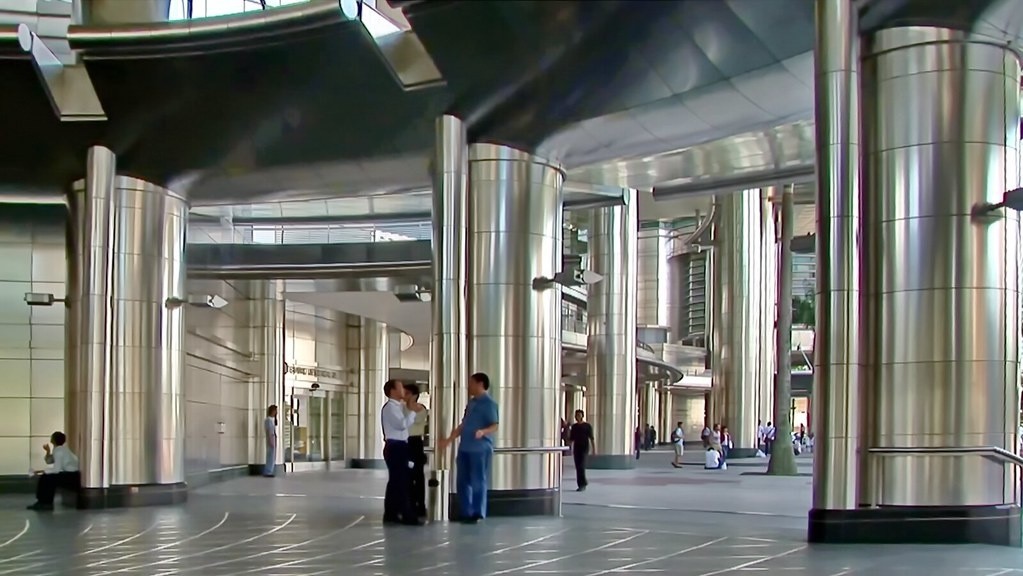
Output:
(792, 437), (801, 455)
(709, 423), (721, 463)
(566, 410), (595, 491)
(800, 423), (806, 441)
(644, 424), (657, 451)
(26, 431), (81, 513)
(700, 425), (710, 449)
(756, 419), (772, 456)
(436, 371), (499, 524)
(703, 447), (723, 471)
(635, 426), (643, 459)
(262, 404), (279, 479)
(670, 422), (684, 468)
(719, 425), (731, 461)
(380, 379), (428, 528)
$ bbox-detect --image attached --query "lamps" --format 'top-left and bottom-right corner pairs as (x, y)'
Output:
(166, 294), (228, 308)
(24, 292), (70, 305)
(394, 285), (432, 302)
(533, 268), (604, 290)
(790, 232), (815, 254)
(991, 187), (1023, 211)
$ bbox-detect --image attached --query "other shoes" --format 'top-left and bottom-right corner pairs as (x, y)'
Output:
(383, 514), (427, 526)
(671, 461), (682, 468)
(577, 485), (587, 491)
(456, 514), (480, 524)
(264, 473), (276, 478)
(26, 501), (54, 511)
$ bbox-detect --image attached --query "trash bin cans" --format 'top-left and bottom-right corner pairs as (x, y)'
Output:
(423, 446), (435, 514)
(428, 470), (449, 522)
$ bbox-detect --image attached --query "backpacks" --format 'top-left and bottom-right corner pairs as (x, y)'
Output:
(671, 427), (684, 443)
